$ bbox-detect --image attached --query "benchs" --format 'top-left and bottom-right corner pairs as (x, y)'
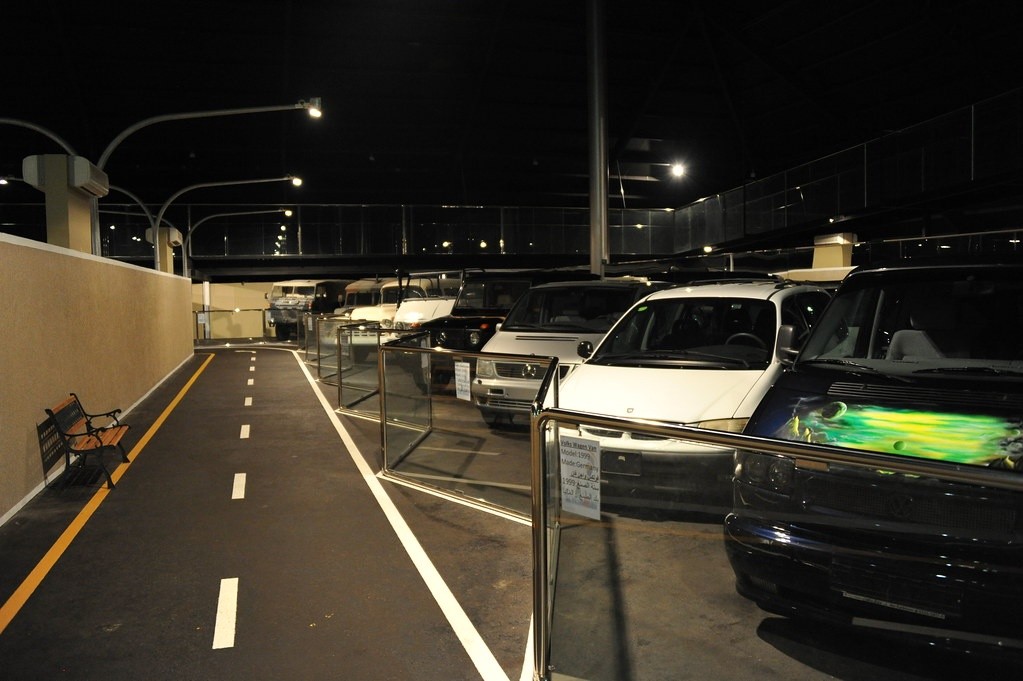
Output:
(42, 392), (131, 490)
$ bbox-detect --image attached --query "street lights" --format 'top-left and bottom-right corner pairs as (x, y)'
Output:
(0, 98), (324, 269)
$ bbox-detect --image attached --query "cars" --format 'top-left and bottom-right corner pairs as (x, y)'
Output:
(542, 270), (833, 513)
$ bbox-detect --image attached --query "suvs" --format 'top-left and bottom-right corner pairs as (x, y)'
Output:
(417, 276), (542, 385)
(263, 274), (465, 354)
(472, 280), (674, 428)
(723, 257), (1023, 649)
